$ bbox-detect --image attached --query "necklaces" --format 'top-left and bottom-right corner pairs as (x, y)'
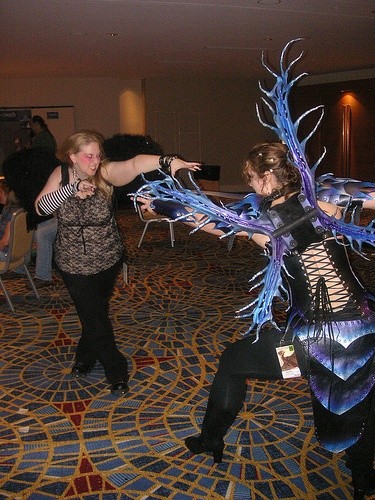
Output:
(72, 168), (92, 183)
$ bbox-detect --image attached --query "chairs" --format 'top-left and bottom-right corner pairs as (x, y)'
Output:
(137, 204), (176, 248)
(193, 165), (220, 203)
(0, 209), (42, 313)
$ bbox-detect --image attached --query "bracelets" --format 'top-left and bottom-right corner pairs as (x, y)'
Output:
(158, 155), (177, 170)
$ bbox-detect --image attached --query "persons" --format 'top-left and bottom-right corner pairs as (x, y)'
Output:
(129, 143), (375, 500)
(36, 130), (202, 396)
(1, 116), (63, 289)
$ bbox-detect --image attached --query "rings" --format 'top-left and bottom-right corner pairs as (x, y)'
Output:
(83, 184), (86, 189)
